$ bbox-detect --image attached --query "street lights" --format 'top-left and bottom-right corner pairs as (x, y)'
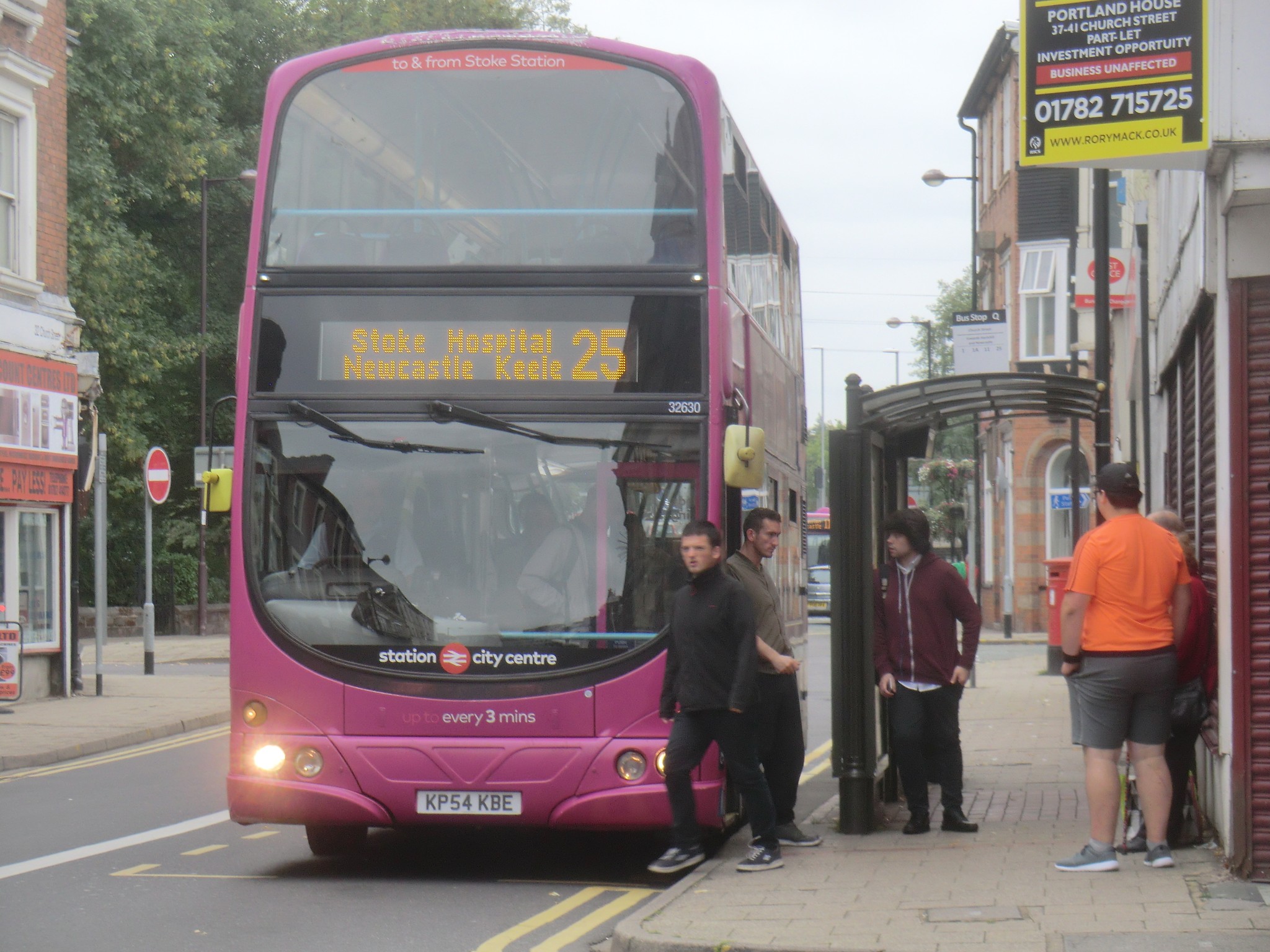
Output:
(886, 317), (935, 504)
(923, 167), (981, 625)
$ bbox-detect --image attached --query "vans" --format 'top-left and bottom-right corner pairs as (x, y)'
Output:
(807, 565), (830, 616)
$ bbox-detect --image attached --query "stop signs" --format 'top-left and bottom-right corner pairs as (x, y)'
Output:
(143, 445), (173, 502)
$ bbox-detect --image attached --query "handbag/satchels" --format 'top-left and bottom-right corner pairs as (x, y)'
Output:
(1172, 678), (1211, 728)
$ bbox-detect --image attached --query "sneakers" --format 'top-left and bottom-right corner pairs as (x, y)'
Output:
(776, 821), (822, 846)
(648, 844), (706, 873)
(1141, 844), (1175, 867)
(1054, 845), (1119, 872)
(1115, 835), (1148, 854)
(902, 815), (930, 834)
(735, 835), (784, 870)
(940, 808), (978, 833)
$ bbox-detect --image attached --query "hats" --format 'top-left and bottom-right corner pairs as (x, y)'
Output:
(883, 509), (931, 556)
(1096, 462), (1143, 508)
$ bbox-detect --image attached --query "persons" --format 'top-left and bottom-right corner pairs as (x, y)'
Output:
(647, 519), (786, 873)
(872, 509), (982, 834)
(298, 506), (423, 580)
(1055, 462), (1190, 872)
(1114, 508), (1210, 853)
(728, 507), (824, 847)
(502, 482), (625, 651)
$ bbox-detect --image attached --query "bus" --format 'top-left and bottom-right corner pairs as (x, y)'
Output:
(806, 506), (832, 571)
(196, 27), (816, 852)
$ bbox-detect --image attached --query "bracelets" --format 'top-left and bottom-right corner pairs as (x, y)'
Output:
(1062, 650), (1081, 664)
